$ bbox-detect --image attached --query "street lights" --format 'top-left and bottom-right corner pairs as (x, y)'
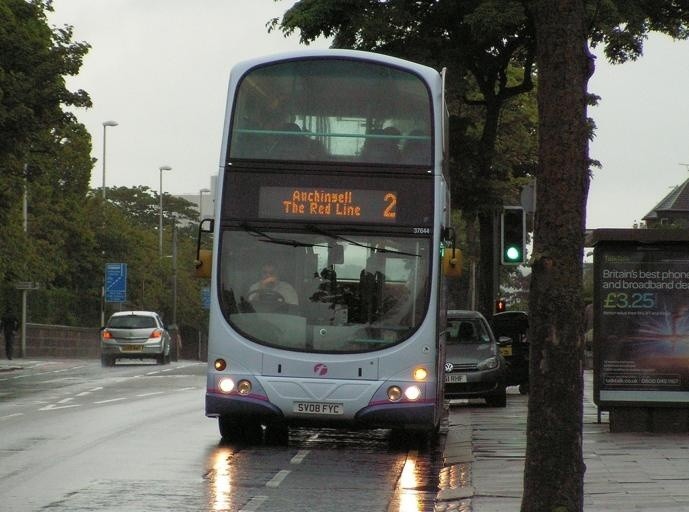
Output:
(101, 119), (120, 328)
(197, 187), (211, 226)
(159, 165), (173, 259)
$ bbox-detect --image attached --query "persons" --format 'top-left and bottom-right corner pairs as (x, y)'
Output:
(247, 262), (299, 305)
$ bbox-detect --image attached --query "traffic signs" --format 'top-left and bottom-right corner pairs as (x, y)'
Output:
(104, 262), (128, 302)
(200, 286), (210, 311)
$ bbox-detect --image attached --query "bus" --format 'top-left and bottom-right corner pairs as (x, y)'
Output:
(204, 48), (451, 448)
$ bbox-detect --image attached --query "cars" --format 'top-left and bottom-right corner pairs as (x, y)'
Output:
(443, 309), (513, 408)
(99, 310), (171, 367)
(494, 310), (529, 396)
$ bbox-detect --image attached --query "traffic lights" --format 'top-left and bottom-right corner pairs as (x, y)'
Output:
(498, 205), (527, 267)
(495, 301), (505, 312)
